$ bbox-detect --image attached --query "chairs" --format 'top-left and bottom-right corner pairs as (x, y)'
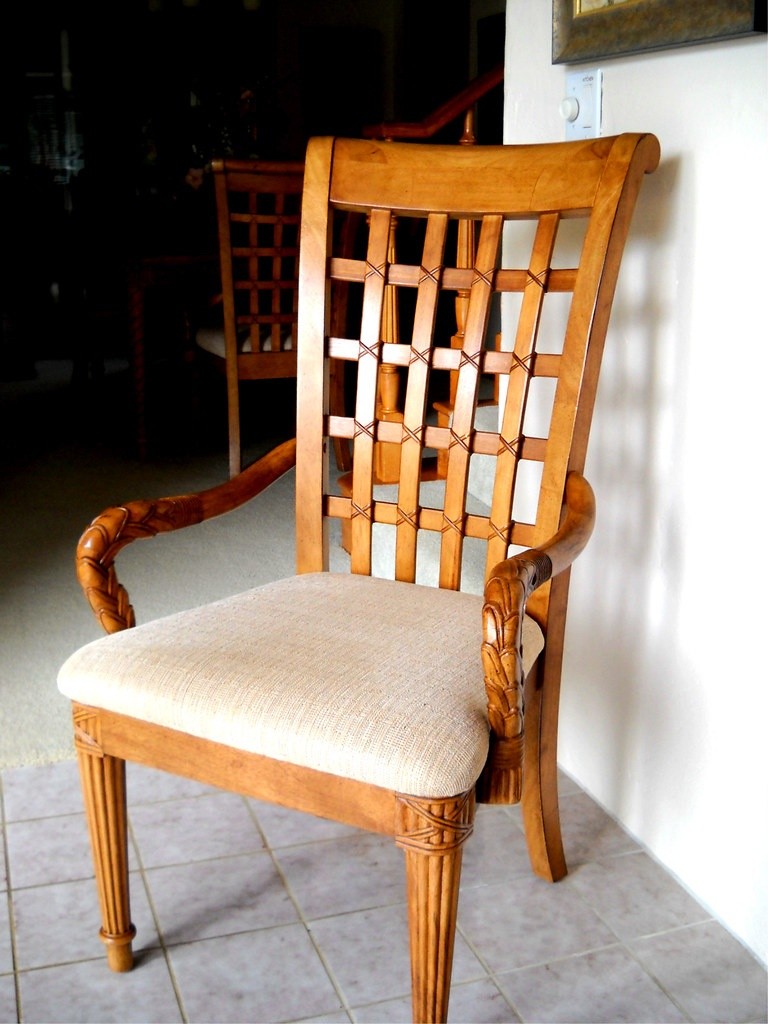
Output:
(46, 123), (665, 1024)
(192, 149), (303, 477)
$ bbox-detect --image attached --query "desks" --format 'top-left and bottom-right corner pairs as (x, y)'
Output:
(127, 240), (252, 467)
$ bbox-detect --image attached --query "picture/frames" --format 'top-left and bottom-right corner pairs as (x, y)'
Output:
(549, 0), (768, 69)
